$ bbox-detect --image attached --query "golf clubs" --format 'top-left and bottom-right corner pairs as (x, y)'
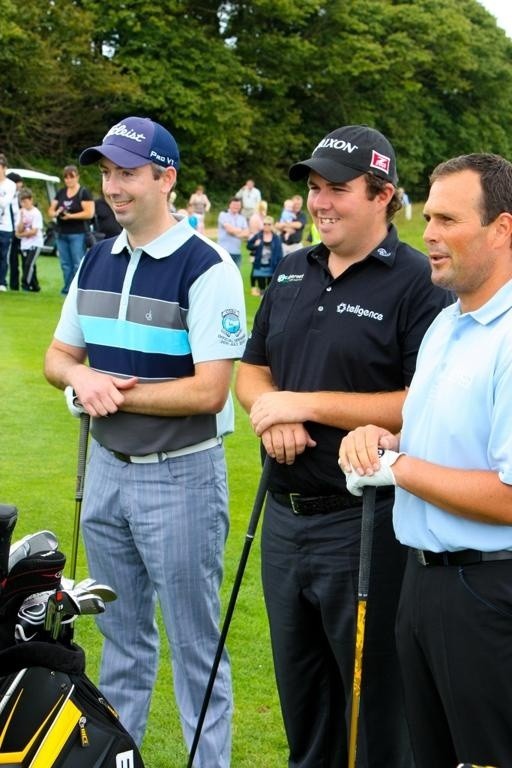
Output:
(8, 530), (117, 648)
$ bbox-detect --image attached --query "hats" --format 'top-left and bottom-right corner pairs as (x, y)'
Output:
(289, 125), (399, 188)
(79, 116), (179, 173)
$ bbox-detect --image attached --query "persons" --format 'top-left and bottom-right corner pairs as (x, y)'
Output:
(169, 185), (211, 234)
(236, 123), (456, 768)
(218, 179), (307, 297)
(0, 154), (43, 293)
(340, 151), (512, 768)
(44, 117), (248, 768)
(47, 165), (123, 293)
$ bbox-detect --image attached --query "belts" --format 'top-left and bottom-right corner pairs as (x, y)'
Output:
(108, 437), (222, 464)
(271, 492), (363, 515)
(413, 550), (512, 566)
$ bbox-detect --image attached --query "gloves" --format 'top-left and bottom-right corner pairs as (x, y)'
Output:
(338, 448), (406, 496)
(64, 386), (83, 417)
